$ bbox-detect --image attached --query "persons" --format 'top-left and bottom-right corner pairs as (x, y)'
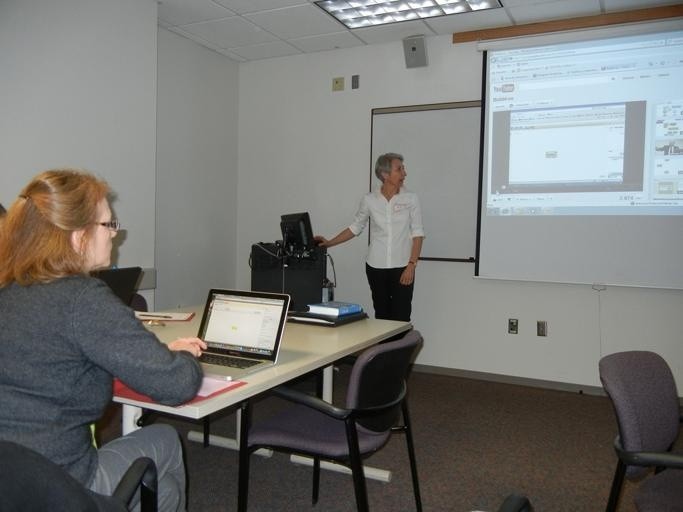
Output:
(656, 140), (683, 156)
(0, 168), (207, 512)
(314, 152), (425, 321)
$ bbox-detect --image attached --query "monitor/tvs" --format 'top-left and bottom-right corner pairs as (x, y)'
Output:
(280, 212), (315, 250)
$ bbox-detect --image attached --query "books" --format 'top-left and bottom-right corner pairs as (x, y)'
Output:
(287, 310), (368, 327)
(306, 300), (361, 317)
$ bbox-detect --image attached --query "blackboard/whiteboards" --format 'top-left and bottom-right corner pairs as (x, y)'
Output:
(368, 100), (483, 263)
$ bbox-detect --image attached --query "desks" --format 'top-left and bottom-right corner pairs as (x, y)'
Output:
(108, 304), (414, 485)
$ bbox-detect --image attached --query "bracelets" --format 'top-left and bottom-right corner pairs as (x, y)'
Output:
(408, 260), (416, 266)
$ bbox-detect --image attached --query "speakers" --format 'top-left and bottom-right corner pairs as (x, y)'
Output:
(401, 35), (428, 70)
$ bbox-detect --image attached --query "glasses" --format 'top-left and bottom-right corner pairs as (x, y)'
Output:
(97, 219), (120, 231)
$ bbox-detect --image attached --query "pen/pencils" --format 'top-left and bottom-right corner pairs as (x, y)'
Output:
(139, 314), (171, 318)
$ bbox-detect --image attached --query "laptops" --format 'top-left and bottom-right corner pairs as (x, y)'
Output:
(196, 288), (290, 382)
(92, 266), (141, 305)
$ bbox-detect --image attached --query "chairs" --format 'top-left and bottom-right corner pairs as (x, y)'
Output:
(0, 434), (158, 511)
(595, 349), (682, 511)
(231, 328), (425, 511)
(86, 262), (148, 315)
